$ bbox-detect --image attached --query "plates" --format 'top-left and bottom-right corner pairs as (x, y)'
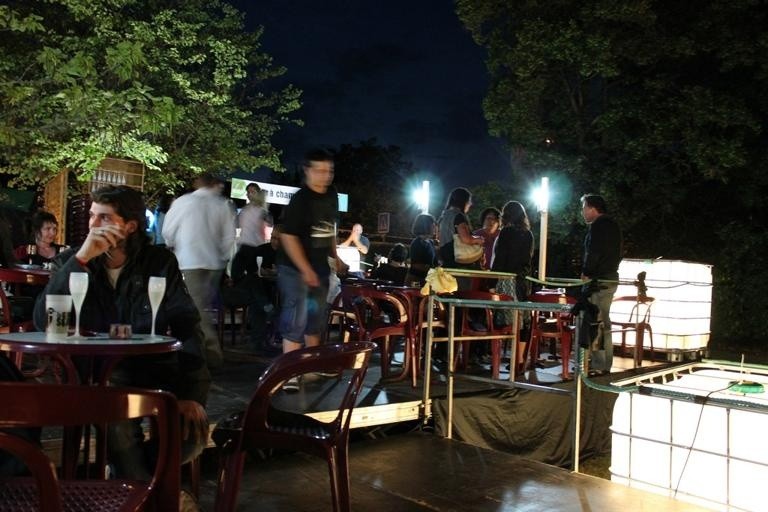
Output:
(12, 262), (45, 270)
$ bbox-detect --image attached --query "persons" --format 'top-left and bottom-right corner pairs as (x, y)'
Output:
(571, 194), (624, 375)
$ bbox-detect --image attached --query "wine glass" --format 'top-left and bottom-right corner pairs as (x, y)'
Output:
(65, 272), (89, 339)
(146, 276), (166, 339)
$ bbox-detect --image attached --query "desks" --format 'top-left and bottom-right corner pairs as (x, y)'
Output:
(4, 332), (203, 511)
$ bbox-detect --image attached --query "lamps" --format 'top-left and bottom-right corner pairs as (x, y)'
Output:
(607, 257), (717, 363)
(608, 357), (768, 506)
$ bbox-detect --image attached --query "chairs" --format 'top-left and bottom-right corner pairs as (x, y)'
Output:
(1, 242), (658, 389)
(211, 341), (379, 512)
(2, 382), (181, 511)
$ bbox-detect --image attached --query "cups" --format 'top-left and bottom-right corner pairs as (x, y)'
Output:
(45, 294), (72, 337)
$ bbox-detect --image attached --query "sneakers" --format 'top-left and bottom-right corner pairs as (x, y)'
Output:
(180, 488), (206, 512)
(423, 352), (495, 365)
(281, 375), (301, 392)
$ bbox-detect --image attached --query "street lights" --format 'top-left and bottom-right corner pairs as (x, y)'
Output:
(532, 175), (550, 282)
(412, 180), (431, 214)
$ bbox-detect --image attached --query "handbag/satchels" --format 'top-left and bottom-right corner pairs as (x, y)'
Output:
(450, 210), (486, 265)
